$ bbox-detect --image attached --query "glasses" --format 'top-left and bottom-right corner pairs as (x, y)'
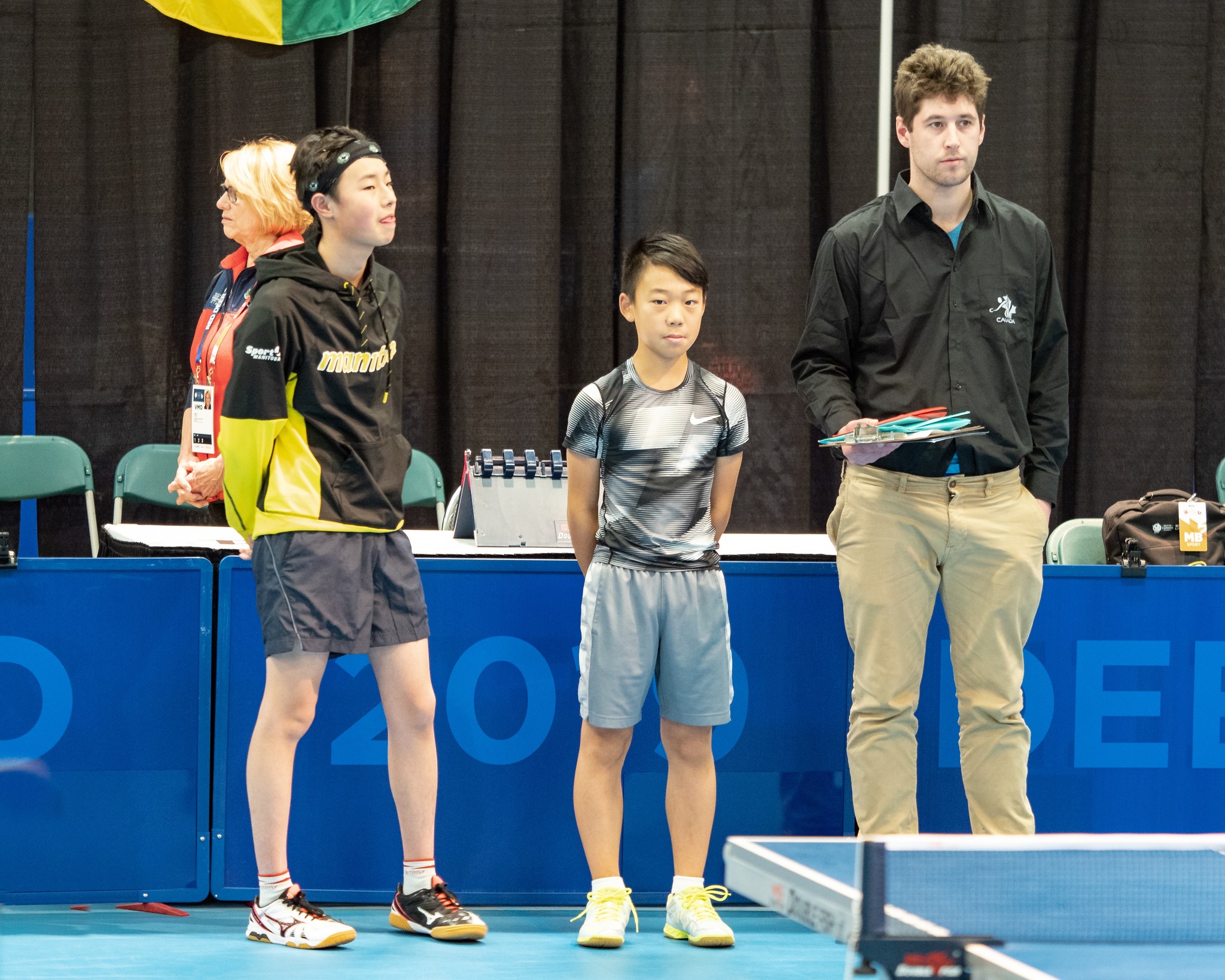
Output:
(219, 184), (244, 203)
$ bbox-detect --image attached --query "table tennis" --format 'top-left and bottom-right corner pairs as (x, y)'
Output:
(1210, 834), (1225, 852)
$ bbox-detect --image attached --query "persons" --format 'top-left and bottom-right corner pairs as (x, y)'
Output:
(562, 234), (751, 947)
(204, 391), (211, 409)
(168, 134), (313, 529)
(218, 125), (488, 948)
(787, 45), (1069, 837)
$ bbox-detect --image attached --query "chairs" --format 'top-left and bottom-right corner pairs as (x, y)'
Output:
(402, 449), (447, 531)
(0, 436), (99, 559)
(1046, 518), (1106, 565)
(113, 444), (208, 524)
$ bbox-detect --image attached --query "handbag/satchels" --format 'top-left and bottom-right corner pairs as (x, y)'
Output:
(1102, 488), (1225, 566)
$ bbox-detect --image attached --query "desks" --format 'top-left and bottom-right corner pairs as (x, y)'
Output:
(724, 832), (1225, 980)
(102, 522), (838, 563)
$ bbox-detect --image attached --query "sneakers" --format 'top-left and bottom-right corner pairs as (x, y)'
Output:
(388, 875), (488, 939)
(663, 886), (735, 945)
(245, 884), (356, 949)
(570, 887), (638, 947)
